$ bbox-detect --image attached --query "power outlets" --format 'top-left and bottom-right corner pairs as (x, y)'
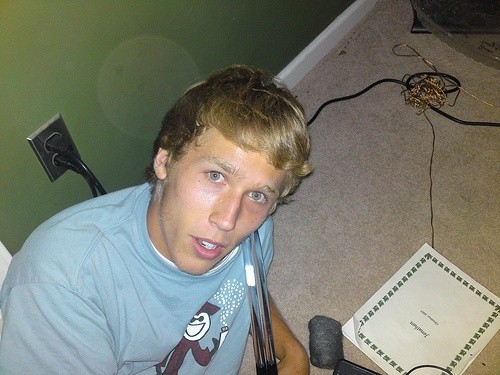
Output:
(27, 113), (80, 183)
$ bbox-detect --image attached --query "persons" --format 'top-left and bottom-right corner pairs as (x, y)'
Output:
(0, 64), (315, 375)
